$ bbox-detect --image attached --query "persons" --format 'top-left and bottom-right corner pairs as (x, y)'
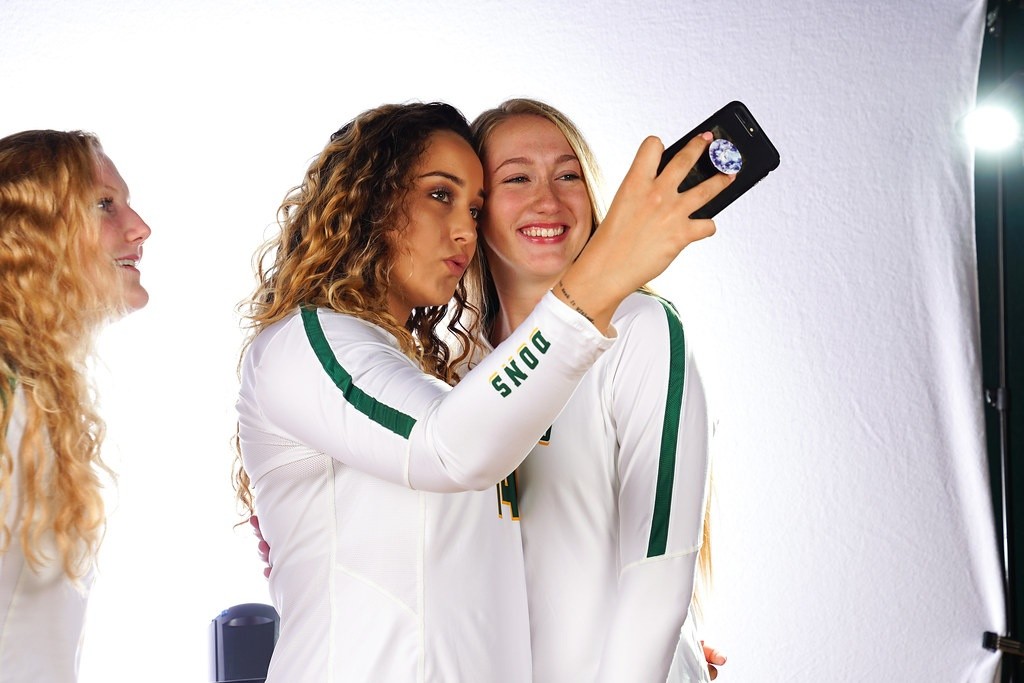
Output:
(0, 127), (152, 683)
(234, 95), (729, 682)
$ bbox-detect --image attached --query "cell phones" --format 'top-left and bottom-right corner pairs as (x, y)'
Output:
(655, 101), (780, 220)
